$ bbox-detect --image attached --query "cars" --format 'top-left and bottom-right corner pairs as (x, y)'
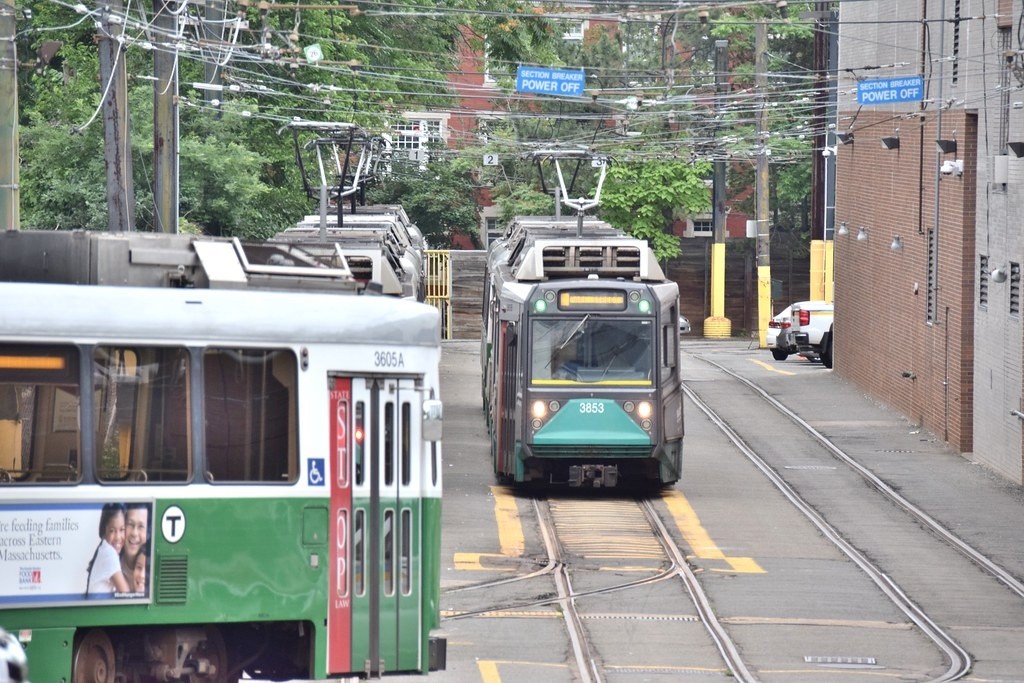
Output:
(791, 301), (833, 369)
(766, 300), (800, 360)
(679, 315), (691, 334)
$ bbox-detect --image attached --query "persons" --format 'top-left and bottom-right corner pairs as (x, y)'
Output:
(119, 503), (149, 593)
(133, 544), (147, 593)
(84, 502), (129, 600)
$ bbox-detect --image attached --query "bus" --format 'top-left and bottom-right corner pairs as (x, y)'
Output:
(0, 234), (445, 683)
(266, 204), (428, 306)
(482, 215), (683, 497)
